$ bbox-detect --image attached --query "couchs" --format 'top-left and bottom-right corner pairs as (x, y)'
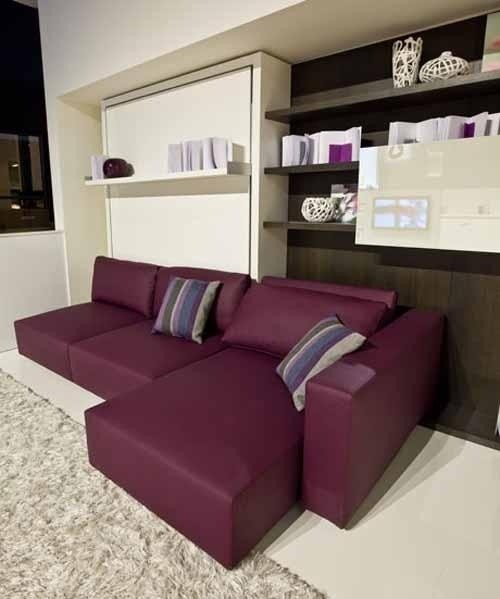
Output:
(13, 252), (450, 569)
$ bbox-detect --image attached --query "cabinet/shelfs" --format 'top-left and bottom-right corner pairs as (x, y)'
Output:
(260, 65), (500, 232)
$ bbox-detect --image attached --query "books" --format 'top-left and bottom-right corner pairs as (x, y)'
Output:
(282, 126), (362, 168)
(388, 111), (500, 145)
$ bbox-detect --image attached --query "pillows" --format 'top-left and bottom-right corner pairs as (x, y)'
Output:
(148, 274), (223, 344)
(272, 313), (367, 413)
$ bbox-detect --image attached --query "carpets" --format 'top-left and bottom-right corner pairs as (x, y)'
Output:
(1, 368), (330, 597)
